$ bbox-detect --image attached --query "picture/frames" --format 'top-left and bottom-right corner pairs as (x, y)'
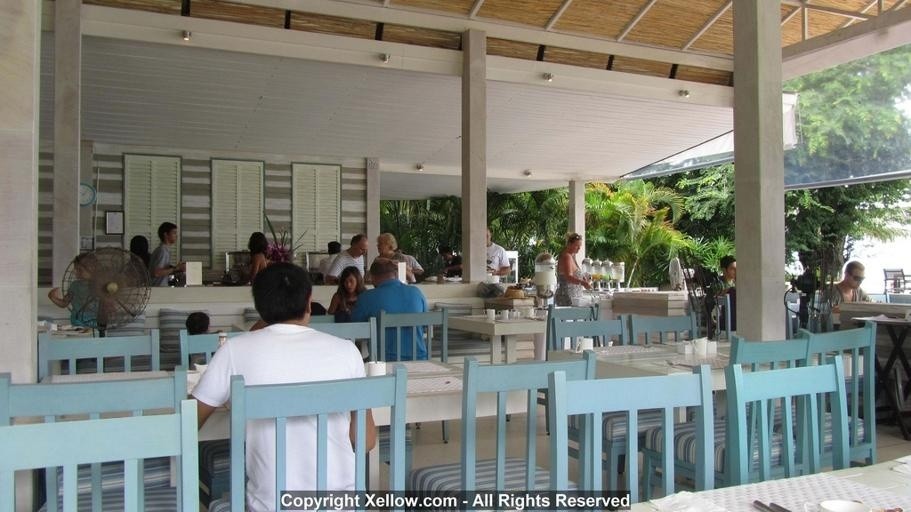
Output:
(105, 211), (125, 235)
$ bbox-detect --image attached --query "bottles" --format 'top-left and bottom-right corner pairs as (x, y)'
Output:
(216, 332), (228, 349)
(504, 287), (525, 298)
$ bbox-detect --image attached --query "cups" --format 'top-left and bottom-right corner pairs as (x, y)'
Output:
(805, 498), (873, 512)
(577, 338), (594, 353)
(367, 361), (388, 376)
(487, 273), (501, 284)
(676, 337), (718, 354)
(484, 308), (522, 322)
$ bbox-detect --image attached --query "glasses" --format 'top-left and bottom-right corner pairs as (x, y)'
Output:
(848, 272), (865, 281)
(568, 236), (582, 243)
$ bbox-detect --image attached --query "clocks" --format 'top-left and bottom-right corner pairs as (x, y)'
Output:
(79, 183), (97, 207)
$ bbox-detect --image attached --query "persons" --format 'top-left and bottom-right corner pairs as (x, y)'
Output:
(185, 262), (378, 512)
(555, 232), (592, 306)
(486, 226), (510, 285)
(185, 312), (222, 370)
(119, 236), (151, 288)
(327, 265), (367, 323)
(315, 241), (340, 286)
(240, 231), (267, 284)
(325, 233), (368, 285)
(438, 246), (462, 277)
(47, 253), (113, 327)
(820, 261), (882, 332)
(375, 233), (423, 284)
(704, 255), (738, 329)
(351, 257), (428, 361)
(144, 221), (186, 288)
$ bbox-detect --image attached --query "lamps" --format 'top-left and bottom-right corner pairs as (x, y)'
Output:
(183, 31), (191, 41)
(380, 53), (392, 63)
(544, 73), (553, 81)
(679, 90), (691, 98)
(417, 162), (424, 172)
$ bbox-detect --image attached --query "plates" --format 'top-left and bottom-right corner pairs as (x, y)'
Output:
(39, 320), (96, 336)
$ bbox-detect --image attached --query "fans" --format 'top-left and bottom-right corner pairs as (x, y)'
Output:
(62, 246), (152, 337)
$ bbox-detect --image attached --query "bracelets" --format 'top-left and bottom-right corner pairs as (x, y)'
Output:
(172, 266), (176, 273)
